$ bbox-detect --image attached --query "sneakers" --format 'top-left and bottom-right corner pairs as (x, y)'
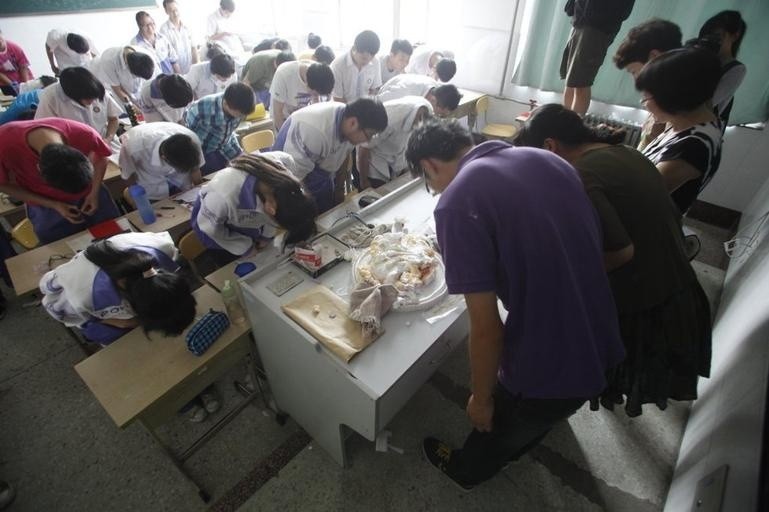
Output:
(199, 385), (222, 414)
(422, 435), (475, 494)
(184, 404), (208, 423)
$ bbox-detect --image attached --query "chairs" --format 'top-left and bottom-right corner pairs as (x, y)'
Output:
(682, 232), (703, 262)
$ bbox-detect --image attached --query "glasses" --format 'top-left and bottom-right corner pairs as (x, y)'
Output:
(360, 128), (380, 141)
(638, 94), (657, 107)
(423, 167), (441, 197)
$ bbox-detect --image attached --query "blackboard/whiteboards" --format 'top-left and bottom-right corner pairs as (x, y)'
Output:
(0, 0), (159, 18)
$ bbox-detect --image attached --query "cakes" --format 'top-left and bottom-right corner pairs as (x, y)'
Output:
(361, 232), (440, 309)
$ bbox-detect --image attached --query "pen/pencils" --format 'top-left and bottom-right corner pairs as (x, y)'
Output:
(156, 214), (175, 217)
(160, 207), (175, 209)
(91, 229), (132, 242)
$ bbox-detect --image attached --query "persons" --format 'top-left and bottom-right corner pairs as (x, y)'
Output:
(612, 16), (684, 151)
(685, 9), (749, 126)
(0, 1), (465, 423)
(635, 47), (724, 222)
(405, 114), (627, 491)
(558, 0), (635, 117)
(508, 103), (715, 418)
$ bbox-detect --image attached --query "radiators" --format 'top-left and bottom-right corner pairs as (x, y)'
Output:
(577, 110), (645, 151)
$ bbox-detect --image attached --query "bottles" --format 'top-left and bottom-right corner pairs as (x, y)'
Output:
(220, 278), (246, 327)
(128, 182), (158, 226)
(123, 96), (139, 127)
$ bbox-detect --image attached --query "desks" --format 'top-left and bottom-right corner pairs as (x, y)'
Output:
(0, 75), (520, 506)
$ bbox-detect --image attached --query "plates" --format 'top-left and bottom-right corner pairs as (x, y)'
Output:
(350, 243), (448, 312)
